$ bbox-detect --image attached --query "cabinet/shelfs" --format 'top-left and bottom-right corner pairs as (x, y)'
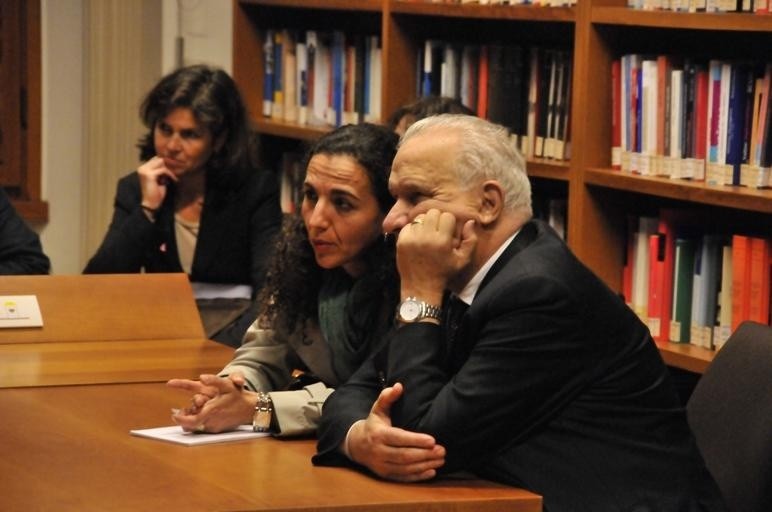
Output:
(230, 0), (772, 376)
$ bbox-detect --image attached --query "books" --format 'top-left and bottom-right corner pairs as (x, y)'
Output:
(262, 28), (381, 125)
(279, 153), (301, 214)
(417, 39), (572, 160)
(612, 53), (771, 187)
(130, 423), (270, 446)
(460, 0), (574, 7)
(629, 0), (772, 14)
(0, 294), (44, 330)
(622, 210), (772, 351)
(535, 198), (566, 240)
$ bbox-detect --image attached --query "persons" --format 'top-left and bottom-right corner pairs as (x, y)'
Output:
(80, 63), (282, 284)
(166, 122), (401, 439)
(0, 183), (52, 277)
(310, 112), (726, 510)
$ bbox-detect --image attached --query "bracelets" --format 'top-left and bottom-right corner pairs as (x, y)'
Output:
(140, 203), (156, 214)
(344, 420), (366, 461)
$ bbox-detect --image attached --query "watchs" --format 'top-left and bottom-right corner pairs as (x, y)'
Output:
(253, 391), (273, 432)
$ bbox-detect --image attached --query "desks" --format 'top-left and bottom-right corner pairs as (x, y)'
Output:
(0, 273), (547, 512)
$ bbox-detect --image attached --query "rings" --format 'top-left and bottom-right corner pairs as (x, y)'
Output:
(195, 424), (205, 432)
